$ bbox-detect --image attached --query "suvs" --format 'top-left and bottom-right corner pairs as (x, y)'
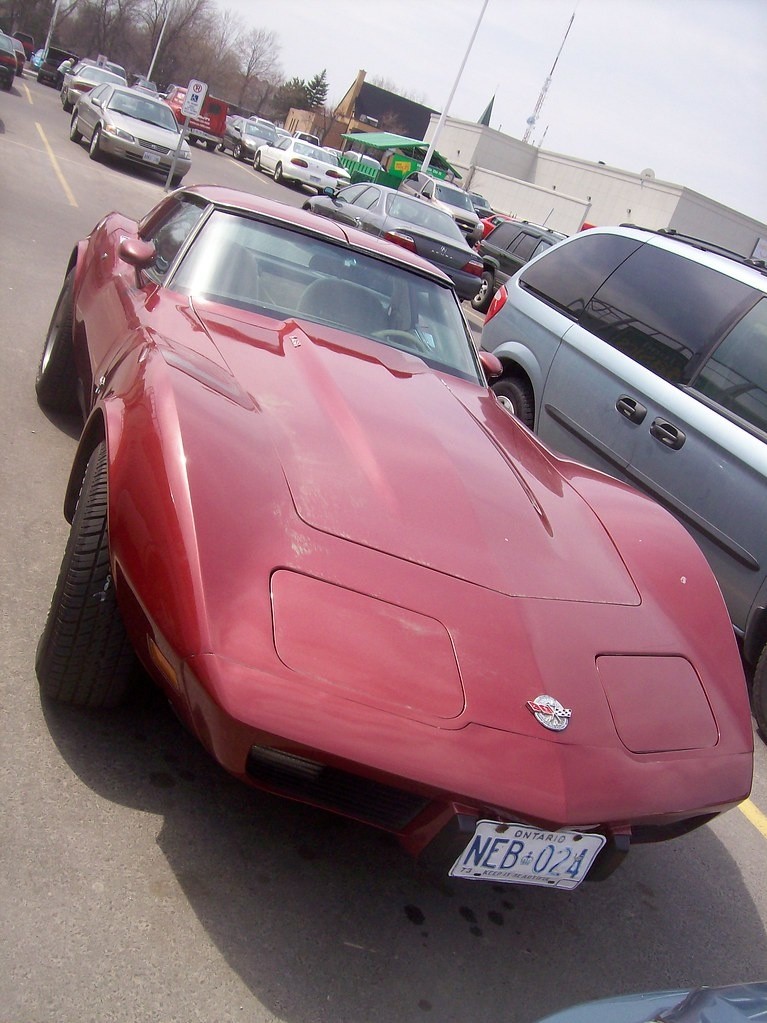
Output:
(478, 223), (766, 748)
(395, 170), (484, 249)
(467, 191), (496, 220)
(469, 219), (571, 314)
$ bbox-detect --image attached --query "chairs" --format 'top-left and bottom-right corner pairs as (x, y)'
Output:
(297, 278), (389, 340)
(308, 151), (319, 159)
(208, 240), (258, 300)
(145, 109), (161, 122)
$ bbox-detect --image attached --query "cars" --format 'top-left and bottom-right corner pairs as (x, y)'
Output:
(302, 181), (485, 303)
(216, 115), (386, 171)
(69, 82), (193, 187)
(253, 136), (352, 196)
(0, 32), (18, 92)
(30, 48), (45, 72)
(479, 214), (514, 240)
(11, 30), (35, 61)
(60, 58), (164, 114)
(9, 36), (27, 77)
(37, 45), (81, 89)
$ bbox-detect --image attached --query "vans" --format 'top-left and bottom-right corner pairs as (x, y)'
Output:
(161, 84), (230, 153)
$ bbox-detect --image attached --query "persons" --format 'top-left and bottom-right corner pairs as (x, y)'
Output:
(52, 56), (74, 91)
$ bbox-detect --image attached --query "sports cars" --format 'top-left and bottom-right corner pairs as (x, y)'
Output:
(34, 183), (753, 890)
